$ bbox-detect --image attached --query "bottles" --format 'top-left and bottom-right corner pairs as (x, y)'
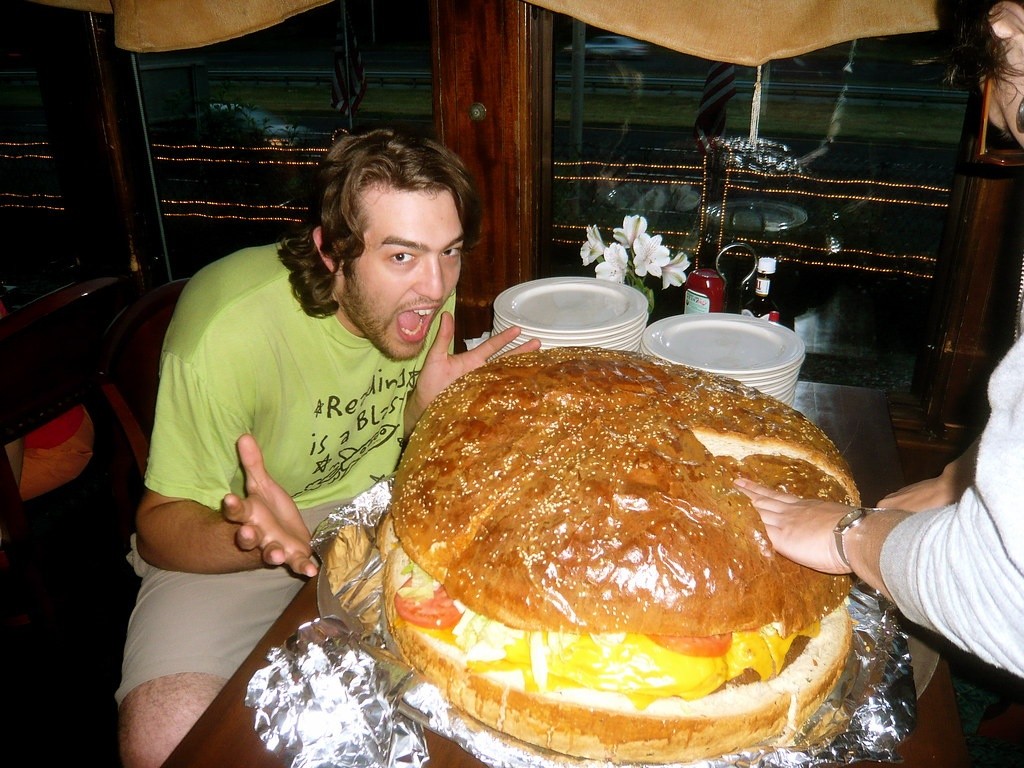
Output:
(742, 256), (787, 307)
(740, 275), (780, 324)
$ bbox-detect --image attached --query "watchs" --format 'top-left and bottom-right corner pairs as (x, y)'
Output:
(833, 507), (885, 567)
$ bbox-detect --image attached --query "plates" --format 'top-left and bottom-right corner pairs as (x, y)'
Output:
(487, 276), (806, 409)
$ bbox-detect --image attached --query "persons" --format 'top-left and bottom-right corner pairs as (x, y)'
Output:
(114, 130), (541, 768)
(734, 0), (1024, 768)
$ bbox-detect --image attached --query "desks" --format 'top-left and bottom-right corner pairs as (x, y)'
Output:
(159, 382), (969, 768)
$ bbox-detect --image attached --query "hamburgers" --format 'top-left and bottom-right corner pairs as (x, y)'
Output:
(376, 347), (860, 762)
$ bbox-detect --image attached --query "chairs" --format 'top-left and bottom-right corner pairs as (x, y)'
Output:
(0, 275), (188, 626)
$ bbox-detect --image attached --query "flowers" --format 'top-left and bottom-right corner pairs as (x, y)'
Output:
(580, 214), (691, 315)
